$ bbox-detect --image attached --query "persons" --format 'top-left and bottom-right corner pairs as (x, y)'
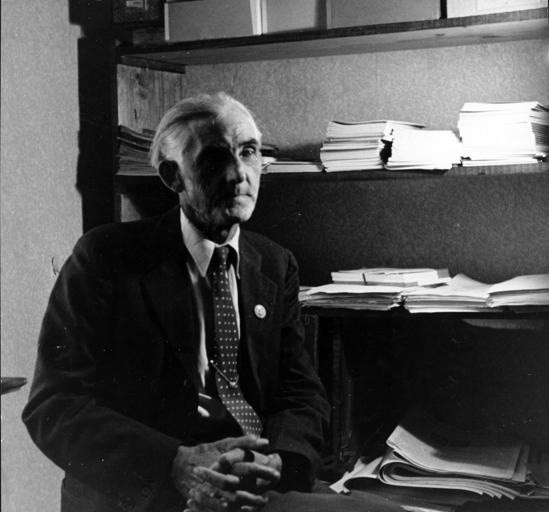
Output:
(21, 92), (331, 511)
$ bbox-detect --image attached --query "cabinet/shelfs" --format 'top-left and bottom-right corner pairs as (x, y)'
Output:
(75, 9), (549, 512)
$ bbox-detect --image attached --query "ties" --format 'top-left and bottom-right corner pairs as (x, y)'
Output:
(210, 245), (264, 438)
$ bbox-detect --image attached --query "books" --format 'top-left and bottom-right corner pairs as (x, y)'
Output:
(259, 142), (323, 173)
(117, 123), (161, 176)
(297, 268), (548, 315)
(328, 425), (549, 501)
(319, 101), (549, 173)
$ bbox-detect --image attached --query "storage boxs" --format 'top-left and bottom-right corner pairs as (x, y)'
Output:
(164, 0), (262, 43)
(326, 0), (440, 31)
(447, 0), (548, 19)
(262, 0), (326, 35)
